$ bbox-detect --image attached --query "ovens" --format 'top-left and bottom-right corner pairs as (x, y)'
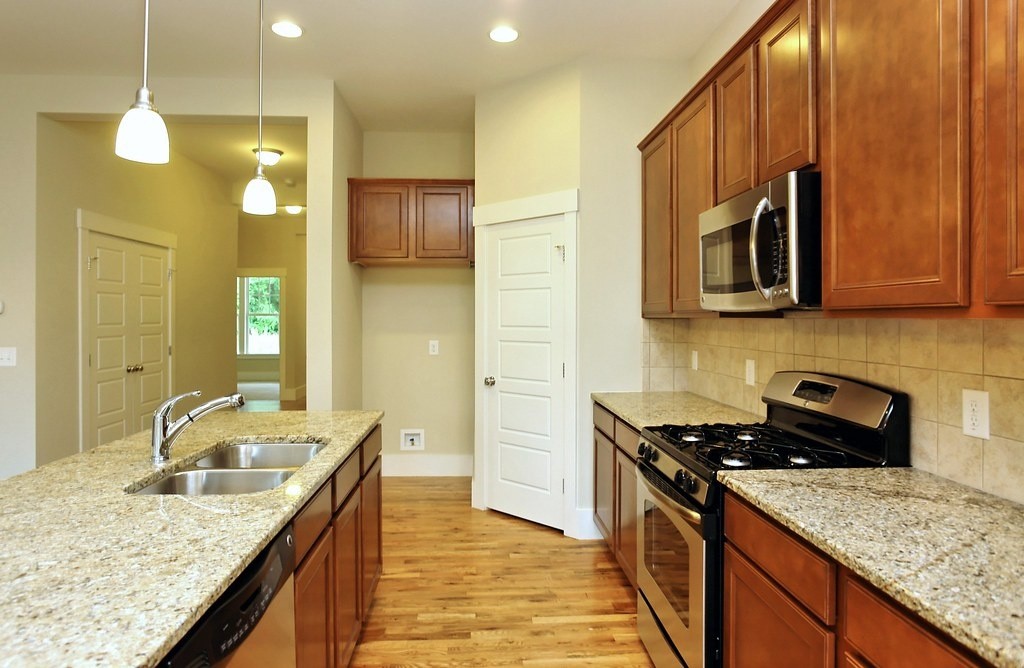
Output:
(637, 458), (725, 667)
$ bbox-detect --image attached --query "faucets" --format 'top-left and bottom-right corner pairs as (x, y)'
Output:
(148, 388), (246, 461)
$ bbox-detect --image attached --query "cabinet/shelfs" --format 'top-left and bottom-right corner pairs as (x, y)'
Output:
(361, 423), (384, 624)
(590, 401), (641, 594)
(714, 0), (817, 207)
(715, 488), (998, 668)
(293, 475), (335, 668)
(346, 177), (476, 268)
(817, 1), (1024, 320)
(333, 445), (363, 668)
(635, 63), (716, 320)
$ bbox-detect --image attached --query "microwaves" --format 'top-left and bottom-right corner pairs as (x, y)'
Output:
(698, 171), (822, 312)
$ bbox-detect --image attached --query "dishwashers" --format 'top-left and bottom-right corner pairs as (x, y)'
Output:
(152, 524), (297, 668)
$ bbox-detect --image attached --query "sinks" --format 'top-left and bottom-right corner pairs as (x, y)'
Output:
(122, 433), (333, 496)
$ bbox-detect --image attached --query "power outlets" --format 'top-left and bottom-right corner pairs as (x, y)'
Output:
(962, 389), (990, 441)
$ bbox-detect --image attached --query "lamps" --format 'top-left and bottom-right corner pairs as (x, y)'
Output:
(115, 0), (172, 165)
(252, 147), (284, 168)
(241, 0), (278, 217)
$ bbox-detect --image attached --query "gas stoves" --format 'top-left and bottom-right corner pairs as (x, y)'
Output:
(635, 369), (913, 512)
